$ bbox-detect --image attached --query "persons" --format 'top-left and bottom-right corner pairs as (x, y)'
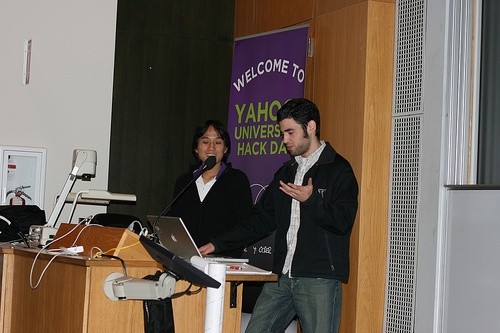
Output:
(195, 98), (359, 333)
(162, 115), (256, 263)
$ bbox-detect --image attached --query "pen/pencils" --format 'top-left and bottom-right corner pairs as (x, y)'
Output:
(228, 264), (244, 271)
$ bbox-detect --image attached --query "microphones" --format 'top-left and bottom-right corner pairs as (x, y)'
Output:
(192, 156), (217, 178)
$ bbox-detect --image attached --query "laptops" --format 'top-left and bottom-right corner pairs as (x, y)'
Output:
(146, 216), (250, 263)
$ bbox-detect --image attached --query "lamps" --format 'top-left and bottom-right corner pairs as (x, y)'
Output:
(28, 149), (97, 247)
(68, 189), (137, 224)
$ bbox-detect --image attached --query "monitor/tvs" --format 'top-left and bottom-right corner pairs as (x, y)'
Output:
(138, 234), (221, 289)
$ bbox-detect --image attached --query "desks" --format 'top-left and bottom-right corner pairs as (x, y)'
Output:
(0, 244), (279, 333)
